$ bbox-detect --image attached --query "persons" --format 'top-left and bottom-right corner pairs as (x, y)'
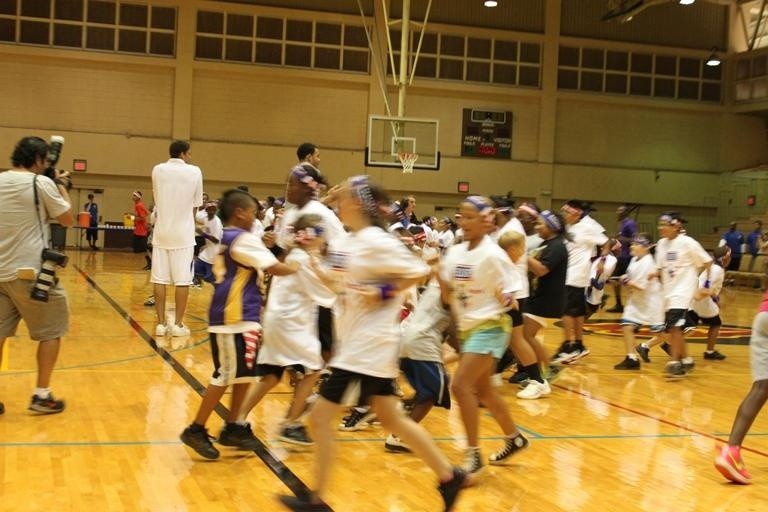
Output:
(84, 193), (100, 251)
(716, 219), (768, 271)
(123, 190), (152, 270)
(713, 289), (768, 485)
(0, 136), (74, 415)
(148, 142), (732, 512)
(131, 215), (135, 221)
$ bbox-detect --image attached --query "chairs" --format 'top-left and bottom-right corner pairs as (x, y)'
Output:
(700, 202), (768, 258)
(724, 254), (753, 291)
(738, 254), (768, 293)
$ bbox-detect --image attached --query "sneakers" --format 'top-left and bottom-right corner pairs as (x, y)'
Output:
(217, 422), (262, 451)
(664, 361), (681, 381)
(551, 338), (590, 365)
(613, 355), (642, 371)
(338, 405), (377, 432)
(672, 357), (696, 378)
(306, 369), (332, 403)
(143, 295), (156, 305)
(172, 322), (191, 338)
(635, 343), (652, 362)
(456, 446), (485, 477)
(715, 439), (754, 484)
(180, 425), (220, 460)
(508, 369), (530, 383)
(703, 351), (725, 360)
(433, 467), (467, 512)
(26, 394), (65, 415)
(155, 323), (169, 336)
(277, 423), (315, 447)
(487, 432), (531, 466)
(385, 433), (413, 453)
(660, 341), (672, 357)
(515, 378), (552, 400)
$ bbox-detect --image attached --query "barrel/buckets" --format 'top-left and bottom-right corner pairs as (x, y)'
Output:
(78, 212), (91, 227)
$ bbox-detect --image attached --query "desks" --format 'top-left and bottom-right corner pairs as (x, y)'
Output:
(71, 224), (137, 251)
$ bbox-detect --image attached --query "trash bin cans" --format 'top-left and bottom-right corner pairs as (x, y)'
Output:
(50, 223), (66, 250)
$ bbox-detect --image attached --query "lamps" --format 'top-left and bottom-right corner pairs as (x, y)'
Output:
(702, 47), (725, 69)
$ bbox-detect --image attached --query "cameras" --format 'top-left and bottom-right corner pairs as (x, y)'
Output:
(39, 136), (72, 193)
(30, 249), (70, 302)
